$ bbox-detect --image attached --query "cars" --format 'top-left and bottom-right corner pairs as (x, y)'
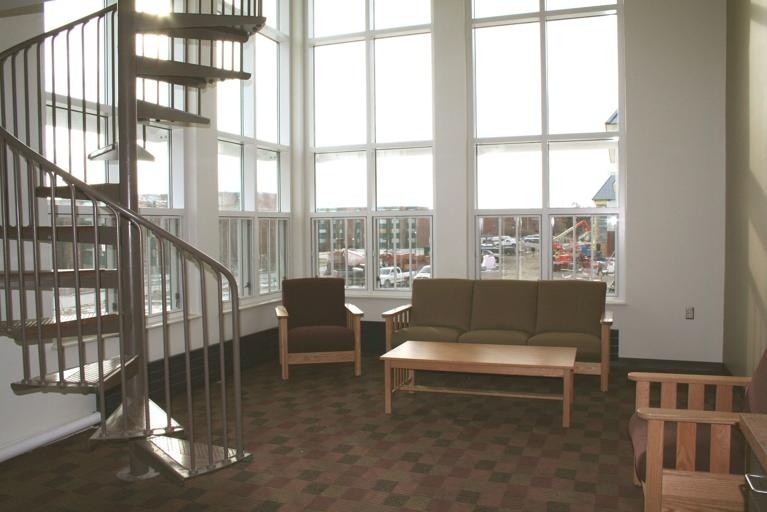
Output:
(480, 233), (538, 255)
(412, 264), (431, 280)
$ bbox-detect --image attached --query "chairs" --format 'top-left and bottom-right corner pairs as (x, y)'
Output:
(627, 344), (767, 512)
(274, 277), (364, 380)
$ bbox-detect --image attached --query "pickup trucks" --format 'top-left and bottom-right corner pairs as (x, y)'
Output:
(480, 250), (499, 263)
(380, 266), (416, 287)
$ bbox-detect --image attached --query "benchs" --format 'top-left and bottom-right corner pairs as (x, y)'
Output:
(381, 279), (614, 393)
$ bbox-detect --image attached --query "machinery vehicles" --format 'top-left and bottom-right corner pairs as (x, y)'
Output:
(552, 220), (597, 272)
(325, 247), (389, 286)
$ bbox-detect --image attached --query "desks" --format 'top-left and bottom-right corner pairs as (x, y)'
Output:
(738, 410), (767, 511)
